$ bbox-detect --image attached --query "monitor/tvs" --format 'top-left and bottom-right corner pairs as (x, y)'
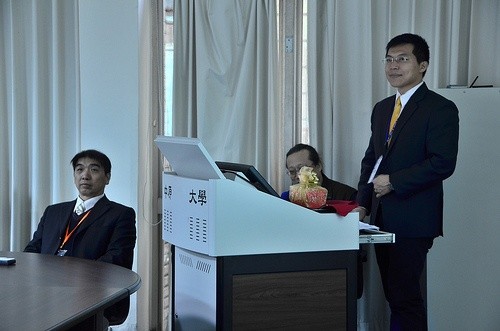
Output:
(214, 162), (280, 198)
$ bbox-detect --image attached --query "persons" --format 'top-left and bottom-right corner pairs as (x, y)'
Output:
(351, 34), (460, 331)
(24, 150), (135, 323)
(285, 143), (357, 201)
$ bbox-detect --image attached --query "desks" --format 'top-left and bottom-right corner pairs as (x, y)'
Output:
(0, 251), (141, 331)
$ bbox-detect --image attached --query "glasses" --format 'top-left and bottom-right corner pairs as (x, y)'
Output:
(384, 56), (409, 65)
(287, 168), (308, 176)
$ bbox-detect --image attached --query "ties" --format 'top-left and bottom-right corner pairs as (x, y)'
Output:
(387, 95), (402, 146)
(77, 202), (84, 215)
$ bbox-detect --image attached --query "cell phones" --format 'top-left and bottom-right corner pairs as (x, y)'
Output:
(0, 256), (15, 265)
